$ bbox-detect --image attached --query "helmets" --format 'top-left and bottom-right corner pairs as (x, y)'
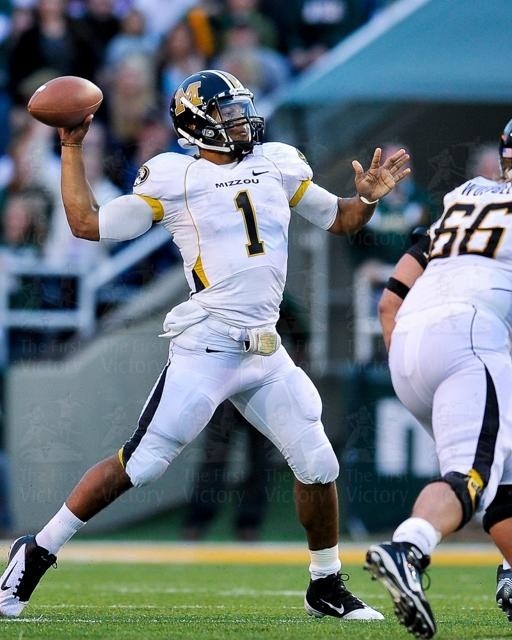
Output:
(169, 70), (265, 156)
(499, 120), (511, 179)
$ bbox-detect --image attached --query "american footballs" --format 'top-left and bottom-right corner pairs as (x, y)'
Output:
(27, 76), (103, 128)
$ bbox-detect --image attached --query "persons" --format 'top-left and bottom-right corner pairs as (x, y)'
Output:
(1, 2), (502, 549)
(0, 67), (415, 624)
(366, 115), (512, 638)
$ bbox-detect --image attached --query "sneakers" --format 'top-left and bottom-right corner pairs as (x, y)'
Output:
(0, 535), (56, 618)
(365, 542), (436, 639)
(305, 574), (383, 621)
(497, 565), (512, 623)
